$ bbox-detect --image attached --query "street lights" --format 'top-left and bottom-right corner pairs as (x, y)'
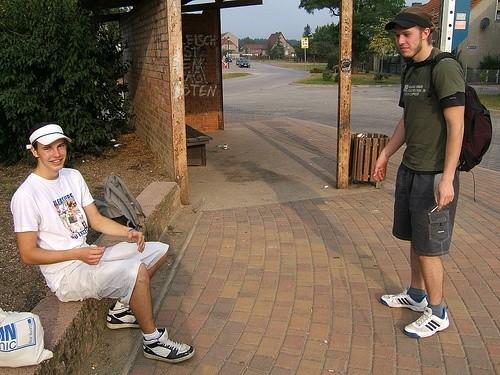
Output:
(227, 36), (230, 69)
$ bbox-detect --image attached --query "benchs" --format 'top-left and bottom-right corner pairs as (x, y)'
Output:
(186, 124), (213, 166)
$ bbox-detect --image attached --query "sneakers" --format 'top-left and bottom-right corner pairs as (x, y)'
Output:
(107, 305), (143, 328)
(381, 289), (430, 312)
(403, 306), (449, 338)
(142, 328), (194, 363)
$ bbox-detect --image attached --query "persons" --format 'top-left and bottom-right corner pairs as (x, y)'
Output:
(372, 7), (467, 338)
(9, 121), (196, 363)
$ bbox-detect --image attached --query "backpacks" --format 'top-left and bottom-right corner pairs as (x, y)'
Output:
(93, 173), (146, 229)
(402, 52), (493, 170)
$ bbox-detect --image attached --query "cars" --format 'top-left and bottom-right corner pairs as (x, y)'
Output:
(239, 59), (250, 69)
(236, 59), (241, 66)
(225, 57), (232, 62)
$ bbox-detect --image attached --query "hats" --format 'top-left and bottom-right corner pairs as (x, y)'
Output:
(384, 14), (432, 30)
(26, 122), (72, 148)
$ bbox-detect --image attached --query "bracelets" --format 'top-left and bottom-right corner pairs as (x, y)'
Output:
(128, 228), (133, 232)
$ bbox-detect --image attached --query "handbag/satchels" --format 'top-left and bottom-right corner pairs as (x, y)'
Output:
(0, 309), (53, 367)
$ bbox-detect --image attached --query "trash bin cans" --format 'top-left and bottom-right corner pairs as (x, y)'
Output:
(349, 132), (389, 181)
